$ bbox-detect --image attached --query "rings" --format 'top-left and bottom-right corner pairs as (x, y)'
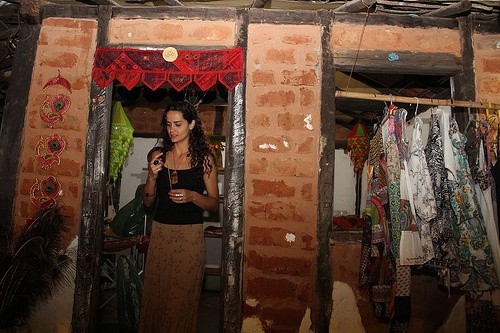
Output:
(175, 193), (183, 200)
(153, 159), (159, 166)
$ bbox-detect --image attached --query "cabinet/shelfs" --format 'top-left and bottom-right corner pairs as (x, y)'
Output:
(331, 216), (363, 289)
(204, 226), (223, 276)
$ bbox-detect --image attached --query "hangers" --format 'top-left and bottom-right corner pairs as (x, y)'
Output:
(384, 94), (499, 134)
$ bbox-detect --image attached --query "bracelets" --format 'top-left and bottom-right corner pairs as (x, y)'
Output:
(142, 190), (156, 197)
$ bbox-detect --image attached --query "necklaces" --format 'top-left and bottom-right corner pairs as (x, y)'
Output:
(170, 146), (189, 183)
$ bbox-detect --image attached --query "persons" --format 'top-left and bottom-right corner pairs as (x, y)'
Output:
(140, 100), (219, 333)
